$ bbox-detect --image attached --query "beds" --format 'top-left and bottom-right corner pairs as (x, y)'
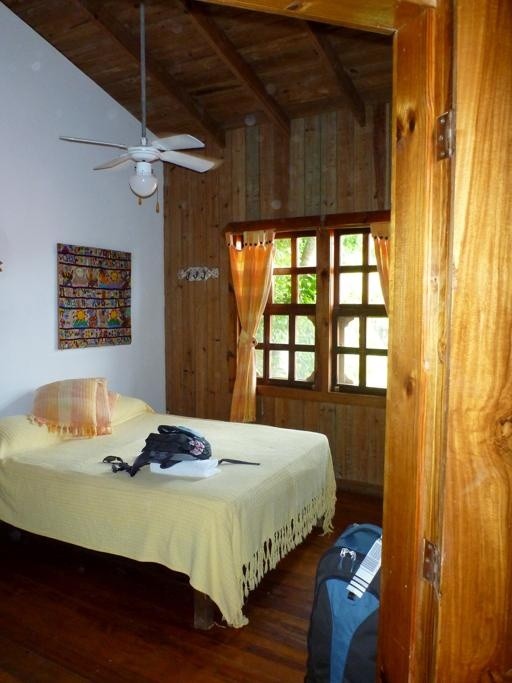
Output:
(0, 413), (327, 631)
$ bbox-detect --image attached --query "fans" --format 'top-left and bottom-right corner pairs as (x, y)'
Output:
(63, 0), (215, 174)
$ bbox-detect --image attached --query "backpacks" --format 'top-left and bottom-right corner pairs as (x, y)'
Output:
(112, 424), (260, 477)
(303, 522), (383, 681)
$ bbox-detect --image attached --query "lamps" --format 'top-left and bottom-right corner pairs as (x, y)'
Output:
(130, 145), (161, 196)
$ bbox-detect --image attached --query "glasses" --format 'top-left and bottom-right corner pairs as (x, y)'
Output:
(103, 455), (123, 463)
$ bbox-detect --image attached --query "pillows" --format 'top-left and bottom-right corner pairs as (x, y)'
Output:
(1, 378), (148, 459)
